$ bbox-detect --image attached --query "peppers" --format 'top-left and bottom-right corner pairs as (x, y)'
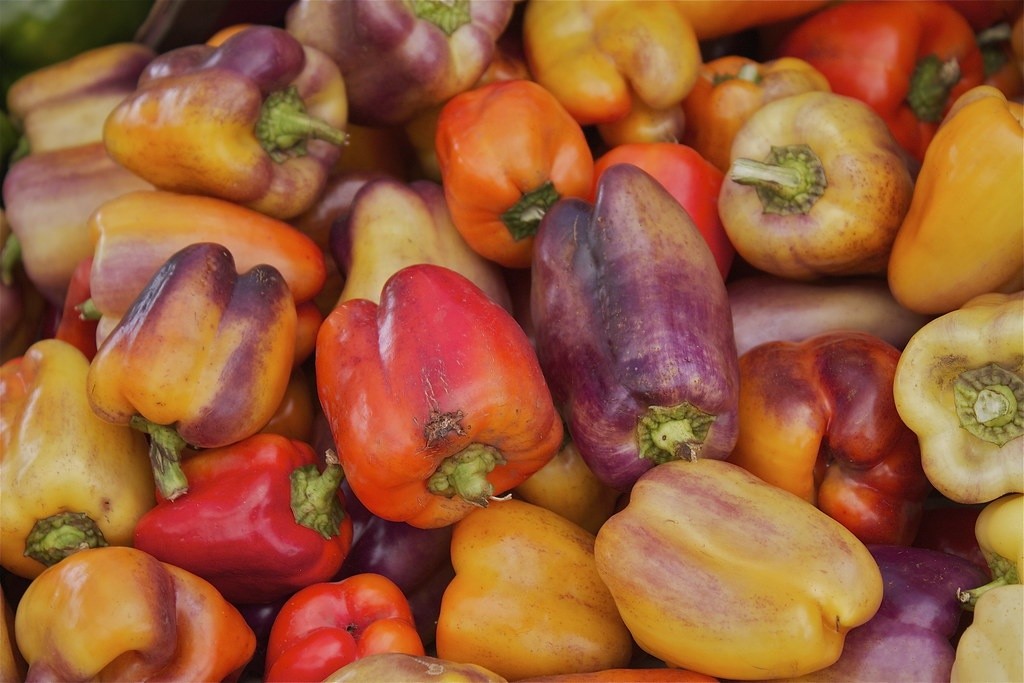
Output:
(1, 3), (1023, 683)
